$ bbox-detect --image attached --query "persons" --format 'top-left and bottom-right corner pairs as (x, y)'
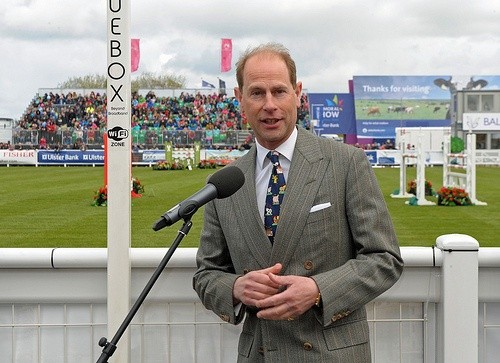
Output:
(354, 139), (412, 151)
(191, 44), (403, 362)
(0, 89), (312, 151)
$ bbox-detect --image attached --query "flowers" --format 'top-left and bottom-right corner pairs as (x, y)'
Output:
(408, 179), (432, 188)
(91, 187), (107, 206)
(436, 187), (471, 205)
(132, 177), (145, 195)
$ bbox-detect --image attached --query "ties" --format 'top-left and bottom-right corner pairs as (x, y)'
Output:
(264, 152), (287, 245)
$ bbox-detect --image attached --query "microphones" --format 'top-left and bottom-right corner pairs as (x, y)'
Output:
(152, 165), (246, 232)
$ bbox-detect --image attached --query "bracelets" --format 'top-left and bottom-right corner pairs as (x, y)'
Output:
(315, 292), (323, 308)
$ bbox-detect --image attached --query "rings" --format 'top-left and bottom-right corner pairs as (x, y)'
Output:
(287, 315), (294, 321)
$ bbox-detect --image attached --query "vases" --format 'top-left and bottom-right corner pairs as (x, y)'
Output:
(408, 186), (433, 196)
